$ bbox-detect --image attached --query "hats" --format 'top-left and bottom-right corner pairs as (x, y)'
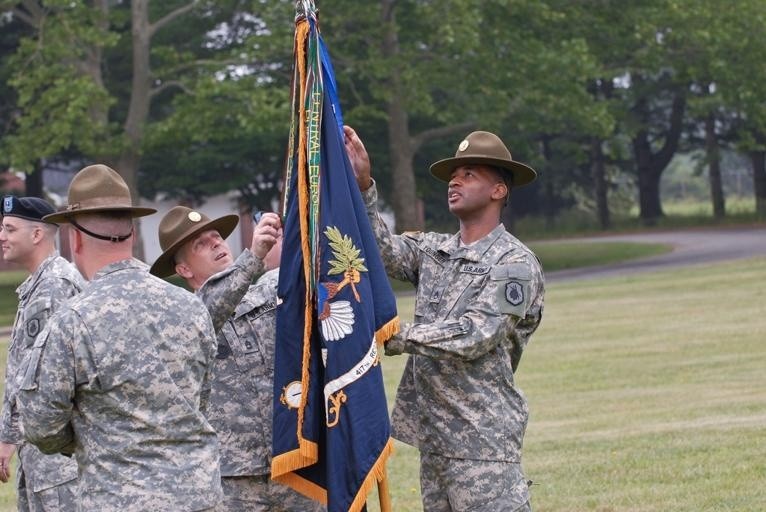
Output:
(428, 130), (537, 189)
(42, 164), (158, 224)
(149, 206), (240, 278)
(0, 195), (59, 225)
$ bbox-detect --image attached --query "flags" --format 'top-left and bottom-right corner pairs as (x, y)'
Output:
(269, 0), (403, 511)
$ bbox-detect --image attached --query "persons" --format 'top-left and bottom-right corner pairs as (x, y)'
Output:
(148, 203), (325, 512)
(1, 194), (89, 511)
(18, 164), (223, 512)
(344, 125), (546, 510)
(256, 218), (283, 292)
